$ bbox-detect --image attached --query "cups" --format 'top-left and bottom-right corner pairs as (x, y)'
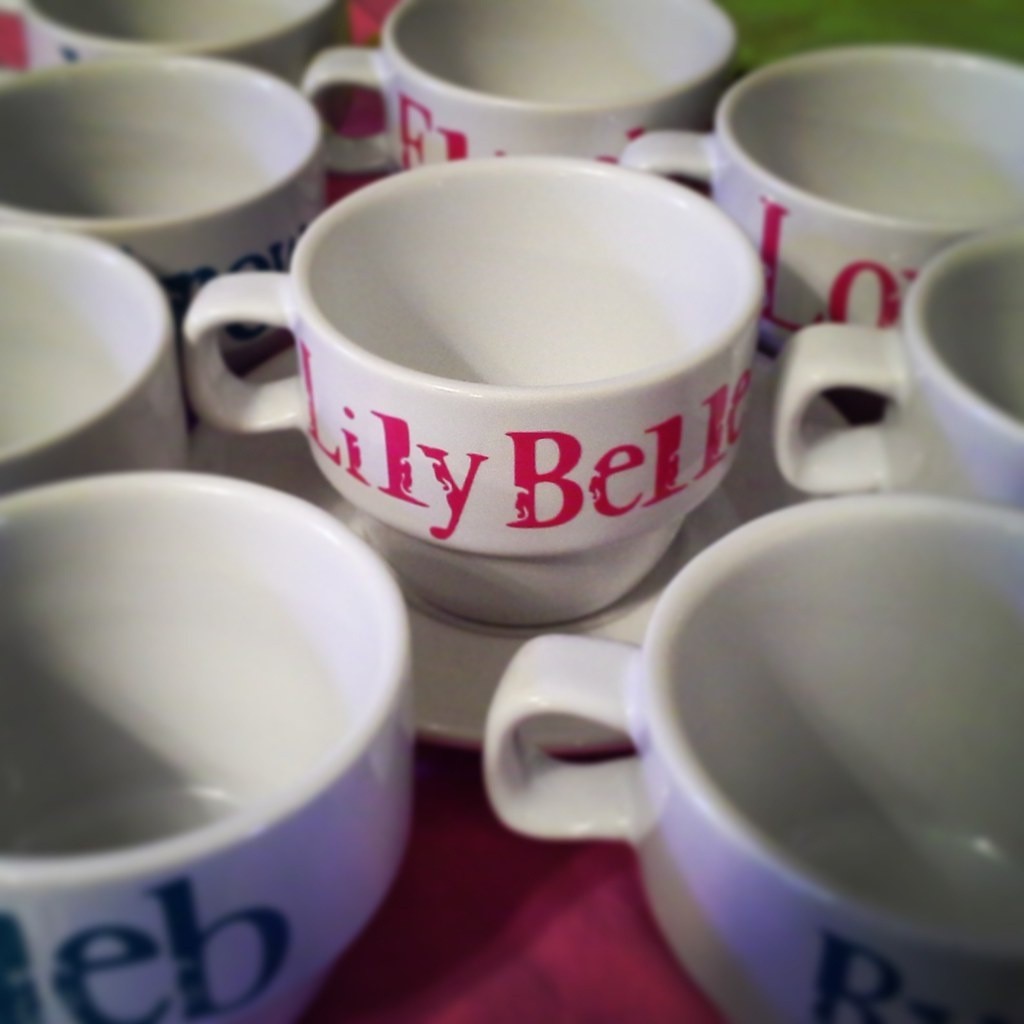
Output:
(0, 55), (325, 407)
(0, 226), (189, 493)
(484, 493), (1024, 1024)
(772, 229), (1024, 510)
(0, 464), (418, 1024)
(0, 0), (349, 126)
(301, 0), (739, 183)
(181, 154), (765, 626)
(620, 43), (1024, 373)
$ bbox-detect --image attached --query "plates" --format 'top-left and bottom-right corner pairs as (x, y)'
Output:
(189, 342), (853, 752)
